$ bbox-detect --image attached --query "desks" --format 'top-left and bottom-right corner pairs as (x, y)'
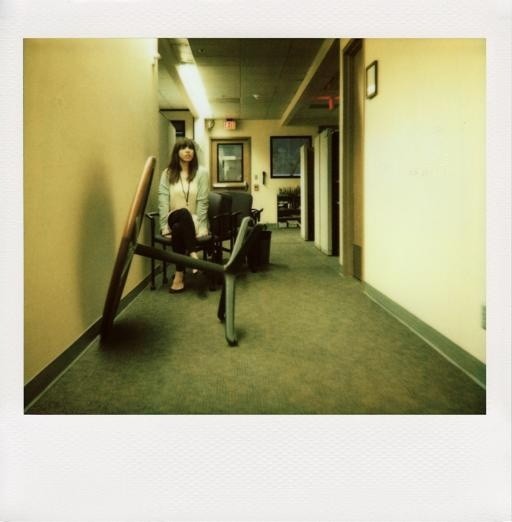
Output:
(101, 156), (254, 346)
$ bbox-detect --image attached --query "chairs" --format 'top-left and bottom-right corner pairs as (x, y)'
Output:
(145, 192), (263, 291)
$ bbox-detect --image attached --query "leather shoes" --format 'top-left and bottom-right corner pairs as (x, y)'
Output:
(170, 274), (184, 293)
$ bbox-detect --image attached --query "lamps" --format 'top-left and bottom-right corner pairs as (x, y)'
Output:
(224, 119), (236, 130)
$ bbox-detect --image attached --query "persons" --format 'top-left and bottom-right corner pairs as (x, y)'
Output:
(156, 137), (214, 294)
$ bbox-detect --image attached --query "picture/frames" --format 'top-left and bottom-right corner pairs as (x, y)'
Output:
(366, 61), (377, 99)
(211, 141), (249, 188)
(271, 136), (312, 178)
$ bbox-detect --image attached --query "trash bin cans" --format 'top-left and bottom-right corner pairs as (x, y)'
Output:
(247, 231), (271, 273)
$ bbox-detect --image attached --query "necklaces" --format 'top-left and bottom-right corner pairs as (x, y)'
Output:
(179, 176), (191, 207)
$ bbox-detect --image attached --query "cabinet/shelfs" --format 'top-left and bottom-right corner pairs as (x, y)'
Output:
(277, 195), (301, 228)
